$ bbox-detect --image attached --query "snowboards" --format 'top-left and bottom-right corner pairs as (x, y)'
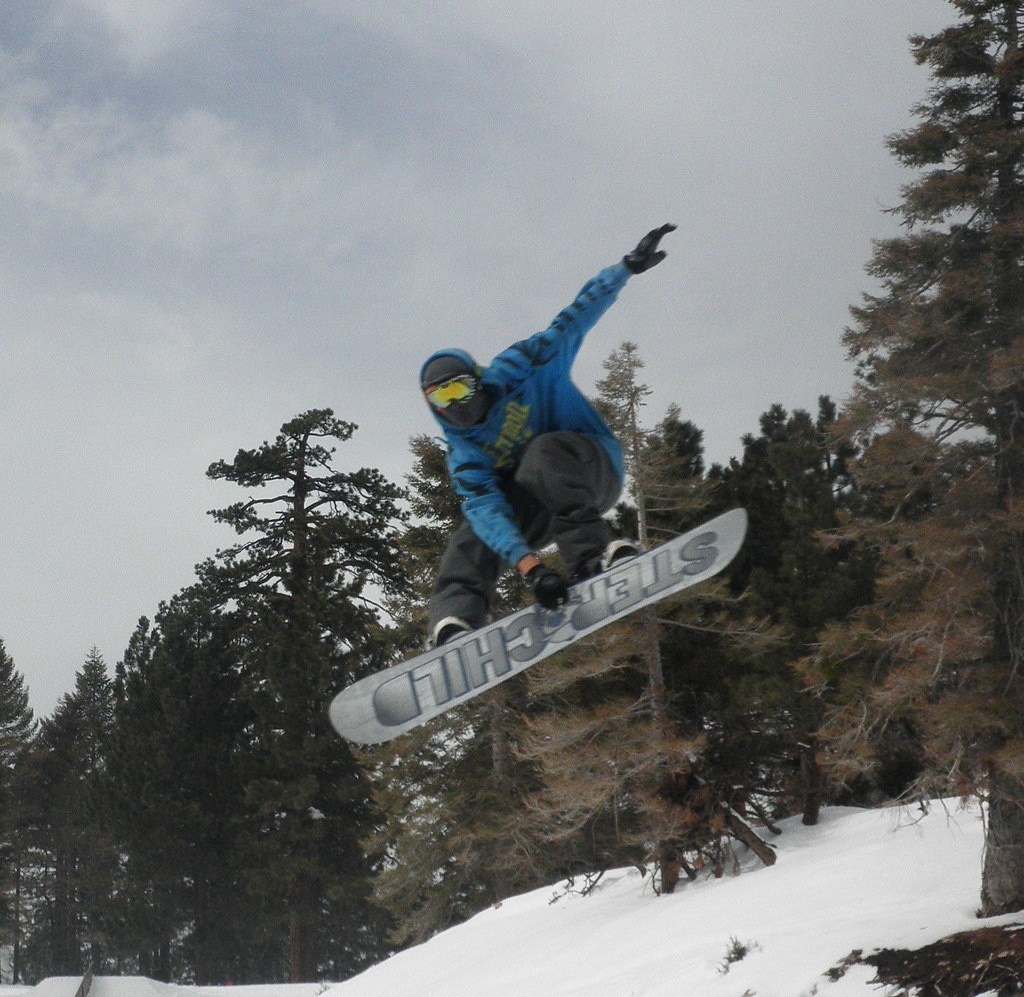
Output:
(327, 506), (751, 746)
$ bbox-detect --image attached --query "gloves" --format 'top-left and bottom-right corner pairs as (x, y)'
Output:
(525, 564), (575, 610)
(624, 222), (677, 274)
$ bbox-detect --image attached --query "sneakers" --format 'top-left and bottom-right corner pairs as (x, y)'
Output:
(600, 540), (641, 571)
(425, 616), (474, 652)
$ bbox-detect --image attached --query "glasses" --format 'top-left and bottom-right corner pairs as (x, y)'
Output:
(426, 374), (478, 407)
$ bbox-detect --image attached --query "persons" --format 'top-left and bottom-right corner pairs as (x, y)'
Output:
(418, 224), (679, 646)
